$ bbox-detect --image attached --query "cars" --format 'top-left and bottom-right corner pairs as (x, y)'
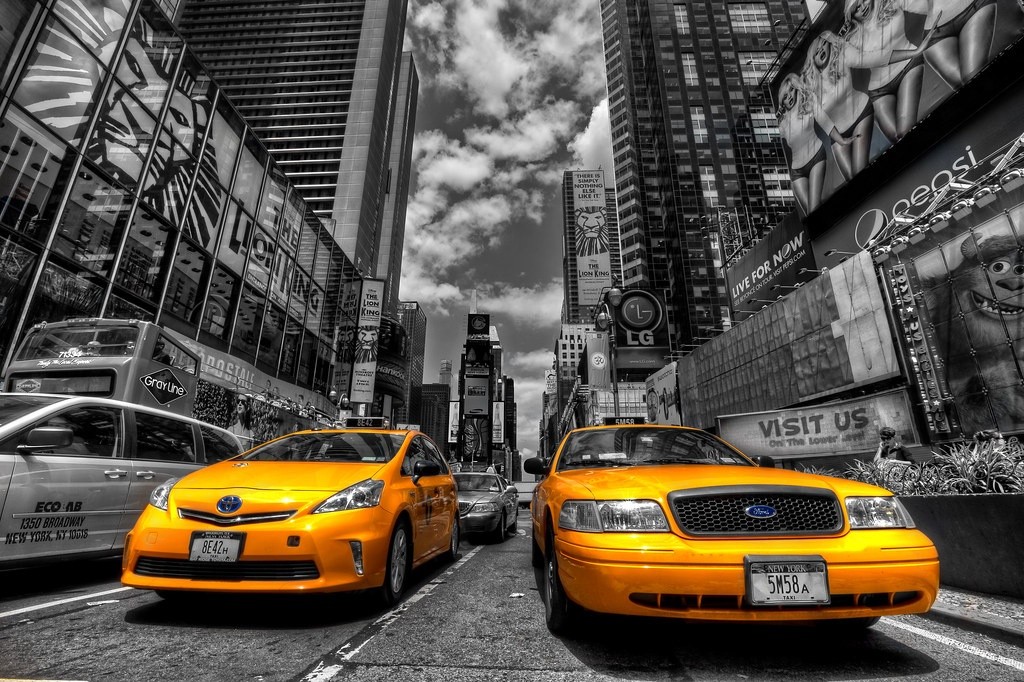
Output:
(452, 465), (521, 541)
(523, 419), (939, 639)
(120, 415), (456, 611)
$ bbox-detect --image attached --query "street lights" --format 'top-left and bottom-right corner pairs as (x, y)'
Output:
(584, 274), (625, 425)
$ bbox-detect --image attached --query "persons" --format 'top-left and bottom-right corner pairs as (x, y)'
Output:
(39, 340), (172, 365)
(775, 0), (1001, 217)
(193, 376), (342, 451)
(35, 416), (90, 454)
(873, 427), (916, 468)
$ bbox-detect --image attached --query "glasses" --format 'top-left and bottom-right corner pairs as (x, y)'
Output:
(237, 399), (248, 405)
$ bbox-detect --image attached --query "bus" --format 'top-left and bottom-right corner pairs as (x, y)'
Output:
(3, 316), (340, 457)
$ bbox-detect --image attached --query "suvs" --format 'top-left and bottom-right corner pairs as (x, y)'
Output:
(1, 393), (244, 568)
